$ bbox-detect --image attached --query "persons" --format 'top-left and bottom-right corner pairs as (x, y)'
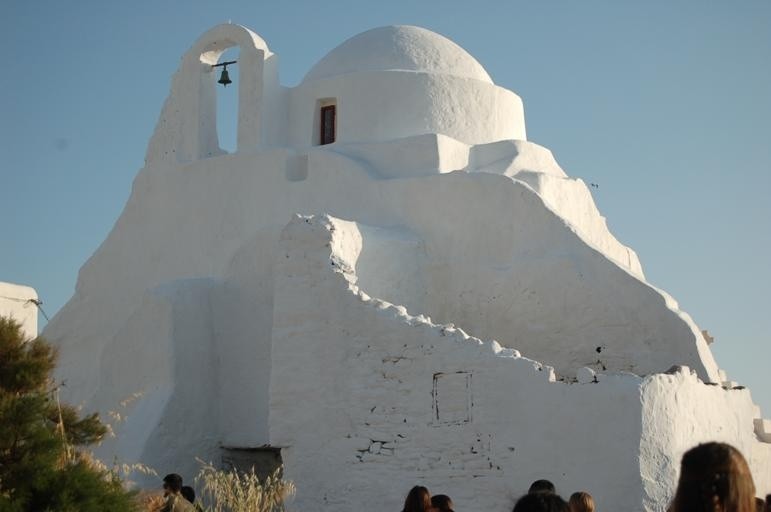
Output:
(403, 485), (454, 512)
(163, 473), (197, 511)
(511, 479), (594, 512)
(665, 441), (771, 512)
